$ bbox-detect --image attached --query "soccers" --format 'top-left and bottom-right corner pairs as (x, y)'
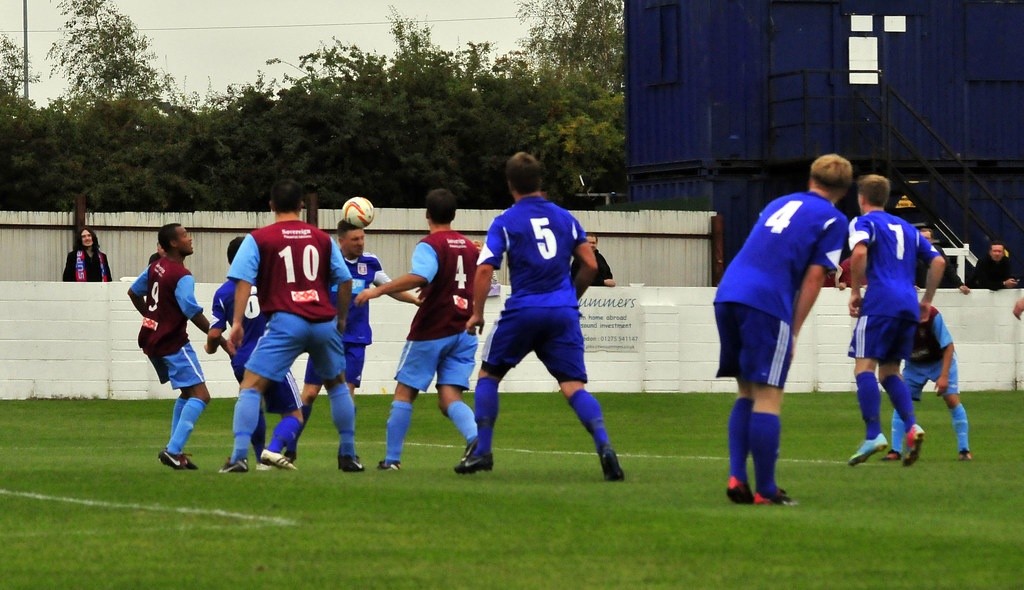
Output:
(342, 197), (375, 228)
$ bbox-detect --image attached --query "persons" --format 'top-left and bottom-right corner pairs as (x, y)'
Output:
(713, 154), (856, 507)
(824, 174), (1024, 466)
(571, 233), (616, 287)
(127, 221), (424, 472)
(63, 227), (112, 282)
(354, 187), (481, 470)
(219, 179), (365, 473)
(473, 240), (498, 286)
(454, 151), (625, 482)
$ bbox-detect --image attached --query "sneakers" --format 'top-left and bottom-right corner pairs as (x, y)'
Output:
(260, 449), (297, 470)
(454, 451), (494, 472)
(377, 460), (400, 470)
(754, 488), (798, 506)
(338, 455), (365, 472)
(727, 475), (754, 504)
(601, 449), (625, 482)
(255, 461), (272, 471)
(882, 450), (902, 460)
(158, 448), (198, 470)
(848, 432), (889, 465)
(218, 456), (248, 473)
(901, 423), (925, 467)
(959, 448), (971, 461)
